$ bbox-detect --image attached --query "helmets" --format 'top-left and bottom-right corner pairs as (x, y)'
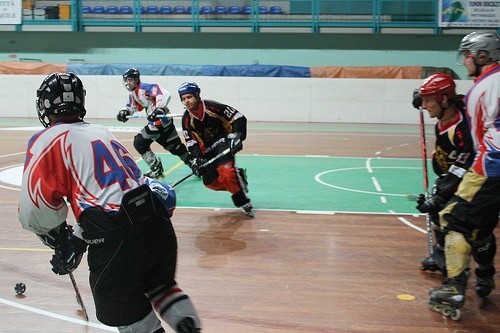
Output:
(460, 30), (500, 61)
(36, 73), (86, 129)
(419, 74), (456, 102)
(179, 83), (200, 98)
(123, 68), (140, 79)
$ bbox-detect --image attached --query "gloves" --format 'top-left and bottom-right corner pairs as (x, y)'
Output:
(147, 108), (165, 121)
(50, 235), (85, 277)
(117, 110), (130, 122)
(230, 135), (242, 154)
(416, 191), (447, 214)
(190, 158), (207, 176)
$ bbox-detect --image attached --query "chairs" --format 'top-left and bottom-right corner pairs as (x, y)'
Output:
(82, 5), (284, 14)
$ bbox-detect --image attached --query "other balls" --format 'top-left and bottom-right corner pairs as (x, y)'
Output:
(14, 282), (26, 295)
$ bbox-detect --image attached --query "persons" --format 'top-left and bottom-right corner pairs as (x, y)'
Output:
(116, 67), (193, 178)
(178, 81), (254, 217)
(414, 72), (476, 279)
(428, 30), (500, 320)
(17, 72), (203, 333)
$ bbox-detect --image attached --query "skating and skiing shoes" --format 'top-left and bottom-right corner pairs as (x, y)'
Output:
(233, 191), (254, 217)
(474, 269), (495, 308)
(144, 158), (163, 180)
(419, 258), (444, 275)
(428, 268), (471, 322)
(238, 169), (249, 192)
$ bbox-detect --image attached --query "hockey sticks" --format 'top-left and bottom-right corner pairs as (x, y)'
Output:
(171, 138), (241, 188)
(68, 272), (89, 322)
(125, 108), (186, 119)
(418, 105), (445, 270)
